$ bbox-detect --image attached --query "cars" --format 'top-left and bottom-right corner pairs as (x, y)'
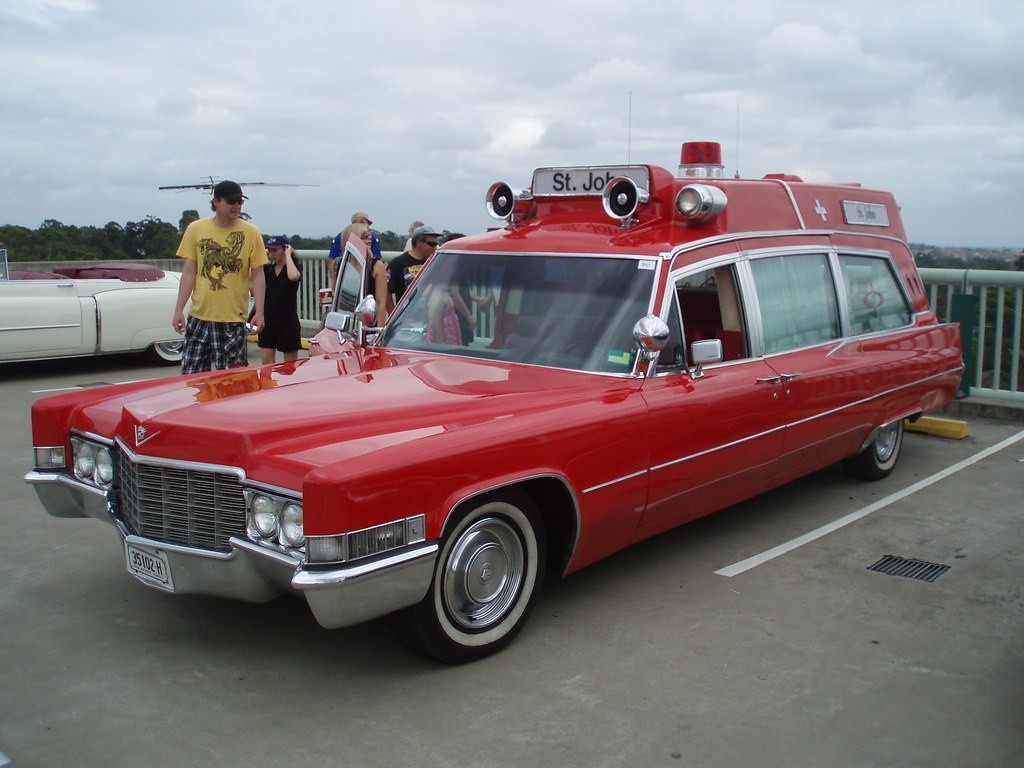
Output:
(22, 140), (967, 666)
(0, 247), (257, 366)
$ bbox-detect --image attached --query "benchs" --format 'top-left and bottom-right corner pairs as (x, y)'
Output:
(1, 270), (68, 281)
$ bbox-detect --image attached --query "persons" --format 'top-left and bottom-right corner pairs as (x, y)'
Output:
(331, 224), (386, 341)
(386, 227), (442, 340)
(172, 180), (268, 375)
(249, 236), (303, 366)
(404, 220), (424, 253)
(425, 270), (462, 345)
(442, 232), (485, 346)
(329, 212), (382, 278)
(480, 265), (523, 316)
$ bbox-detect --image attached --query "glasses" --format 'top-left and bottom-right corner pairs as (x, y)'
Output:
(422, 239), (437, 247)
(226, 199), (244, 205)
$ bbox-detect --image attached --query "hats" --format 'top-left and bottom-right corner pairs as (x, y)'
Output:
(351, 212), (373, 227)
(214, 181), (249, 200)
(265, 236), (288, 249)
(412, 226), (443, 238)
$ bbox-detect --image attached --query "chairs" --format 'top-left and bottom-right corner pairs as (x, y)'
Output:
(503, 313), (546, 353)
